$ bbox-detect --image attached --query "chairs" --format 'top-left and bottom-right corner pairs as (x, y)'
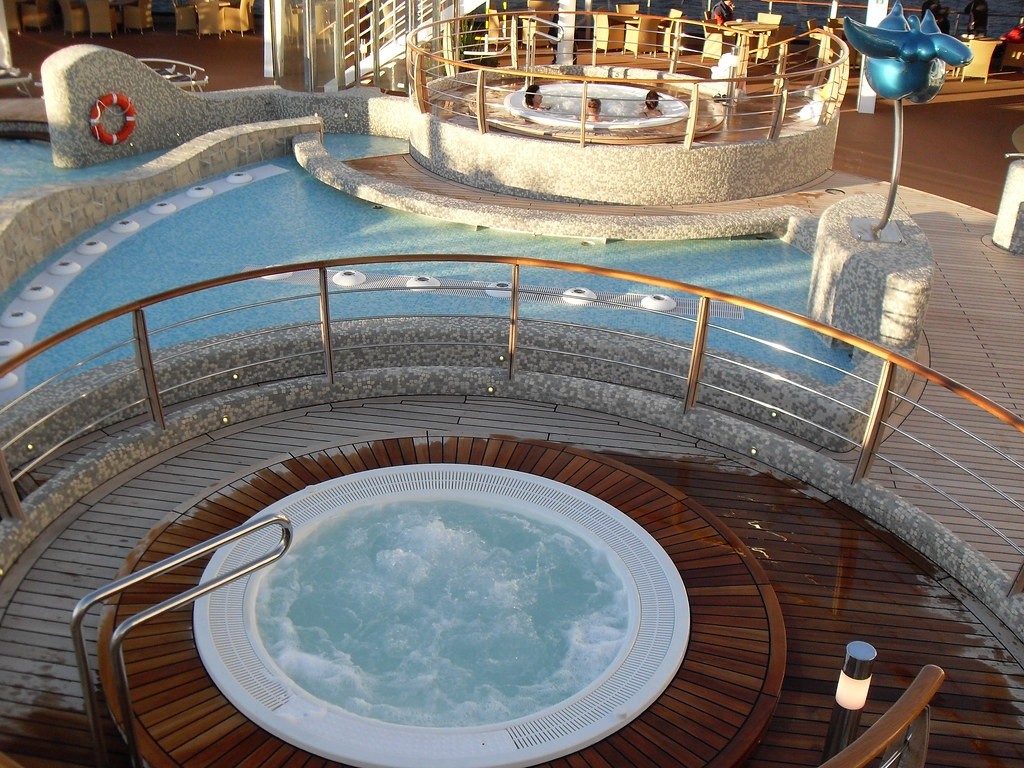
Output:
(592, 4), (687, 57)
(961, 41), (996, 84)
(172, 0), (256, 40)
(59, 0), (155, 40)
(999, 17), (1024, 71)
(486, 0), (556, 50)
(3, 0), (55, 36)
(701, 11), (796, 67)
(805, 18), (861, 70)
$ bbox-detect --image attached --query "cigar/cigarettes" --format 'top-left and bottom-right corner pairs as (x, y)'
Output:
(733, 6), (735, 7)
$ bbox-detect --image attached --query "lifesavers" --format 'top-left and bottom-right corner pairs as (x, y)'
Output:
(88, 92), (136, 144)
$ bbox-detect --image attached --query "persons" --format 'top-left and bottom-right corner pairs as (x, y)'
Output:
(640, 90), (663, 118)
(922, 0), (950, 36)
(966, 0), (988, 35)
(588, 99), (608, 121)
(546, 11), (577, 64)
(525, 85), (546, 111)
(711, 0), (737, 37)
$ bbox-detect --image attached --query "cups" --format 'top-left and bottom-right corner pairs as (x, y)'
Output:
(736, 19), (743, 23)
(968, 34), (975, 39)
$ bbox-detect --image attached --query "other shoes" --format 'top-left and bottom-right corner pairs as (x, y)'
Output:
(712, 92), (727, 105)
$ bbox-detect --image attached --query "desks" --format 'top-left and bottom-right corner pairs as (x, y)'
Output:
(195, 0), (229, 10)
(109, 0), (135, 8)
(724, 20), (779, 61)
(952, 36), (1008, 77)
(613, 16), (639, 25)
